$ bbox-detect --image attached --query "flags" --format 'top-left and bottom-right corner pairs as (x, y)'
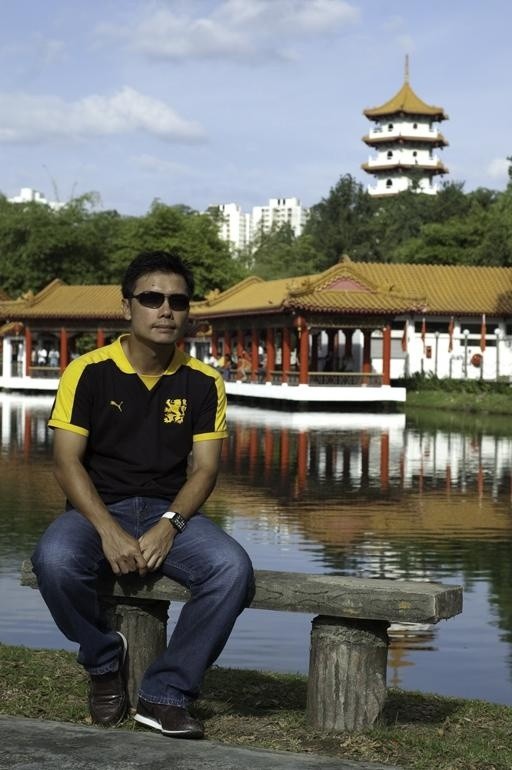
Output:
(401, 318), (409, 354)
(448, 316), (456, 354)
(421, 317), (426, 342)
(480, 313), (487, 352)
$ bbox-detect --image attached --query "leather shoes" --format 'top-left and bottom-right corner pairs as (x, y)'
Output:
(135, 697), (206, 740)
(88, 630), (129, 729)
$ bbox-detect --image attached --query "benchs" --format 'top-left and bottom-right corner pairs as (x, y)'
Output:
(20, 569), (463, 735)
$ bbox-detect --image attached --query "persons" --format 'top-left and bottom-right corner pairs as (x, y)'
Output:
(69, 349), (81, 363)
(48, 345), (60, 377)
(254, 354), (267, 384)
(29, 249), (254, 738)
(295, 350), (355, 385)
(37, 344), (48, 377)
(16, 340), (23, 377)
(202, 350), (252, 382)
(31, 346), (37, 378)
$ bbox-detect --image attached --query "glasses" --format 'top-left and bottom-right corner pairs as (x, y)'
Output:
(128, 290), (190, 312)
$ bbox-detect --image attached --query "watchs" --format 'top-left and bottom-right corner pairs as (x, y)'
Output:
(161, 511), (188, 535)
